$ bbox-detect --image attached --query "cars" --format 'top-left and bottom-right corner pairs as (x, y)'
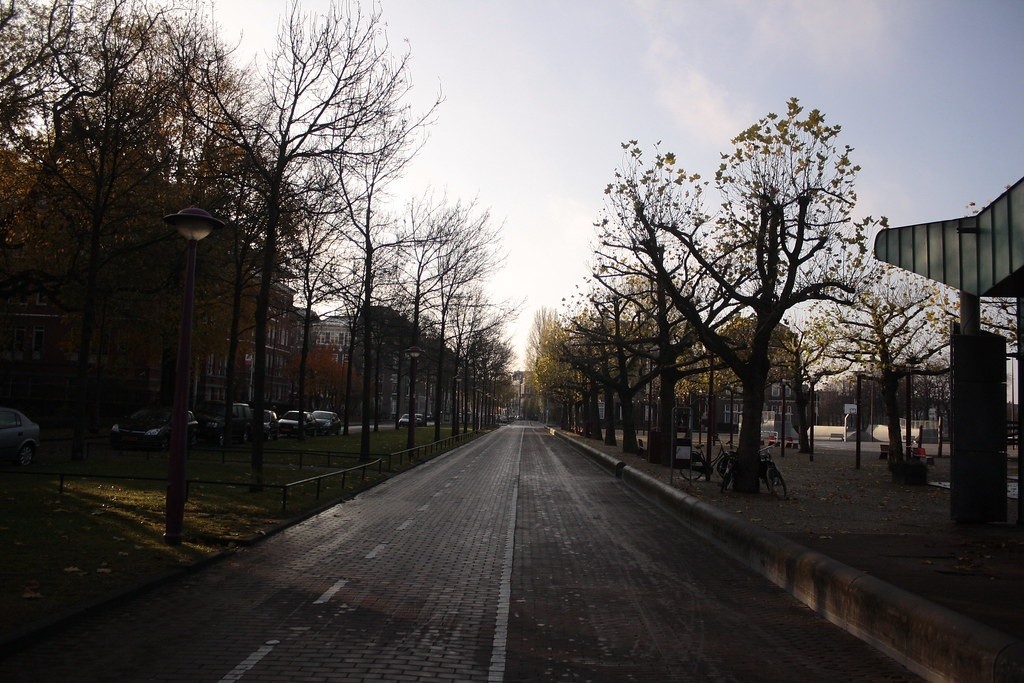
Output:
(249, 407), (280, 441)
(399, 412), (424, 428)
(278, 410), (319, 440)
(312, 408), (341, 436)
(0, 407), (42, 466)
(109, 408), (200, 452)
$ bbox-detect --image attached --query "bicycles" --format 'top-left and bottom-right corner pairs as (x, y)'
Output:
(679, 435), (787, 501)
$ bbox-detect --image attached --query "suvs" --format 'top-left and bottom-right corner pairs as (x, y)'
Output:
(193, 399), (252, 450)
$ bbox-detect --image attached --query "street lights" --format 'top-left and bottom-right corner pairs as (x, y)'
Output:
(855, 374), (869, 470)
(159, 206), (230, 547)
(474, 386), (484, 435)
(723, 384), (733, 450)
(402, 345), (425, 456)
(453, 375), (466, 442)
